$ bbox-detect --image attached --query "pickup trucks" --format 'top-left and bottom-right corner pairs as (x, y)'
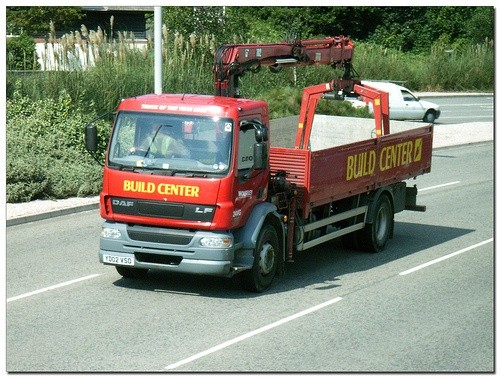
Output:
(343, 78), (440, 124)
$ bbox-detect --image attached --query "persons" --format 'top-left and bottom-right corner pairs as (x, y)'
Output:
(126, 119), (187, 159)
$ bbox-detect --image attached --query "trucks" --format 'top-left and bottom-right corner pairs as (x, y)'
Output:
(84, 34), (438, 295)
